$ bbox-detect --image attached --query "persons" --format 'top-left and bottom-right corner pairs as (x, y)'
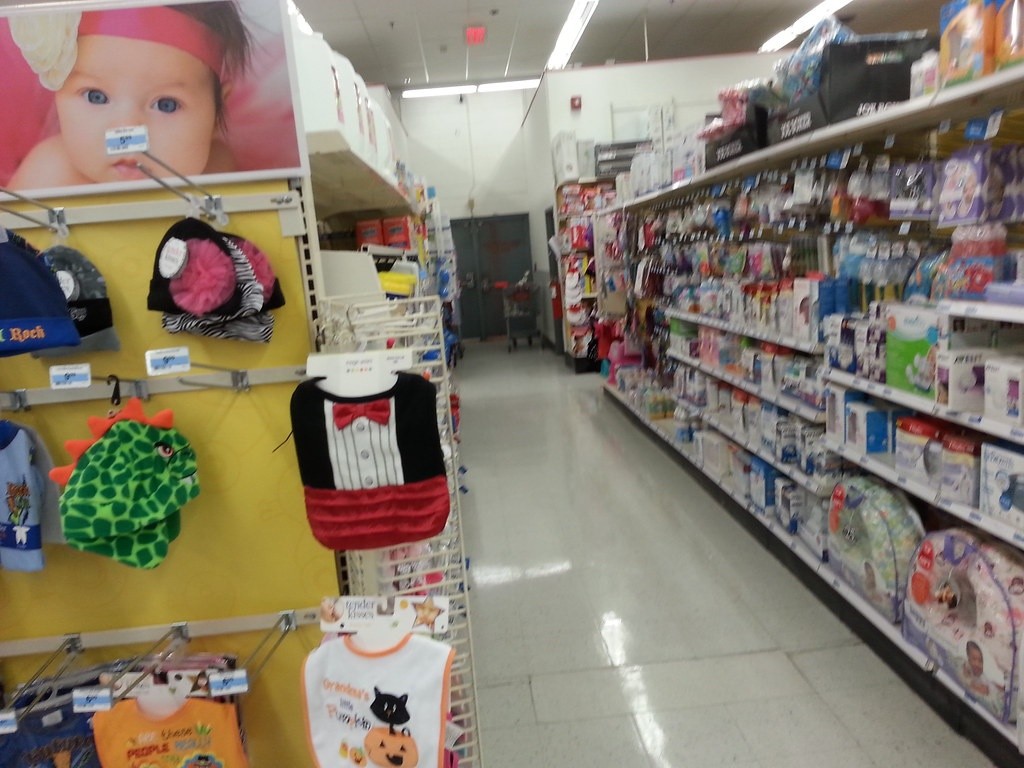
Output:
(963, 175), (975, 208)
(7, 0), (251, 193)
(963, 641), (990, 696)
(989, 184), (1006, 214)
(938, 584), (957, 608)
(862, 562), (884, 602)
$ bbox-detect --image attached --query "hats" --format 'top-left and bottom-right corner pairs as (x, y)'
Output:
(0, 217), (284, 573)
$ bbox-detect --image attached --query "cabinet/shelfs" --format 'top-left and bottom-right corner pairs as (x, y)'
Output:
(560, 1), (1024, 768)
(0, 1), (483, 768)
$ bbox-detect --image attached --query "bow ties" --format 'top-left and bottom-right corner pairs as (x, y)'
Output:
(332, 400), (391, 430)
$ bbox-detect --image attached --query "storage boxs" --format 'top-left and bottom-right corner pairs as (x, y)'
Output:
(766, 89), (828, 146)
(704, 105), (764, 171)
(819, 37), (939, 124)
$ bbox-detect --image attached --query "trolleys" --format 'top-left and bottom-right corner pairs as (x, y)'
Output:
(496, 282), (546, 347)
